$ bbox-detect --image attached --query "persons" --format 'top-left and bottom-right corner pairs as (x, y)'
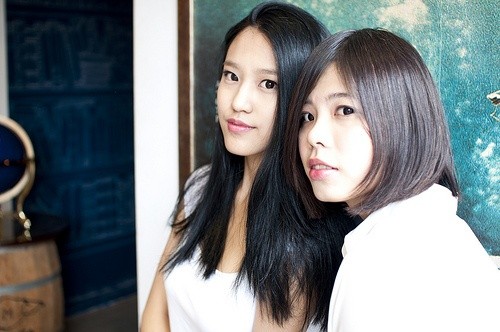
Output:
(140, 2), (333, 332)
(282, 28), (500, 332)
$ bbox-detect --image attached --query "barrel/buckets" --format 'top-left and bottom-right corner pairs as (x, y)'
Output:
(0, 239), (64, 332)
